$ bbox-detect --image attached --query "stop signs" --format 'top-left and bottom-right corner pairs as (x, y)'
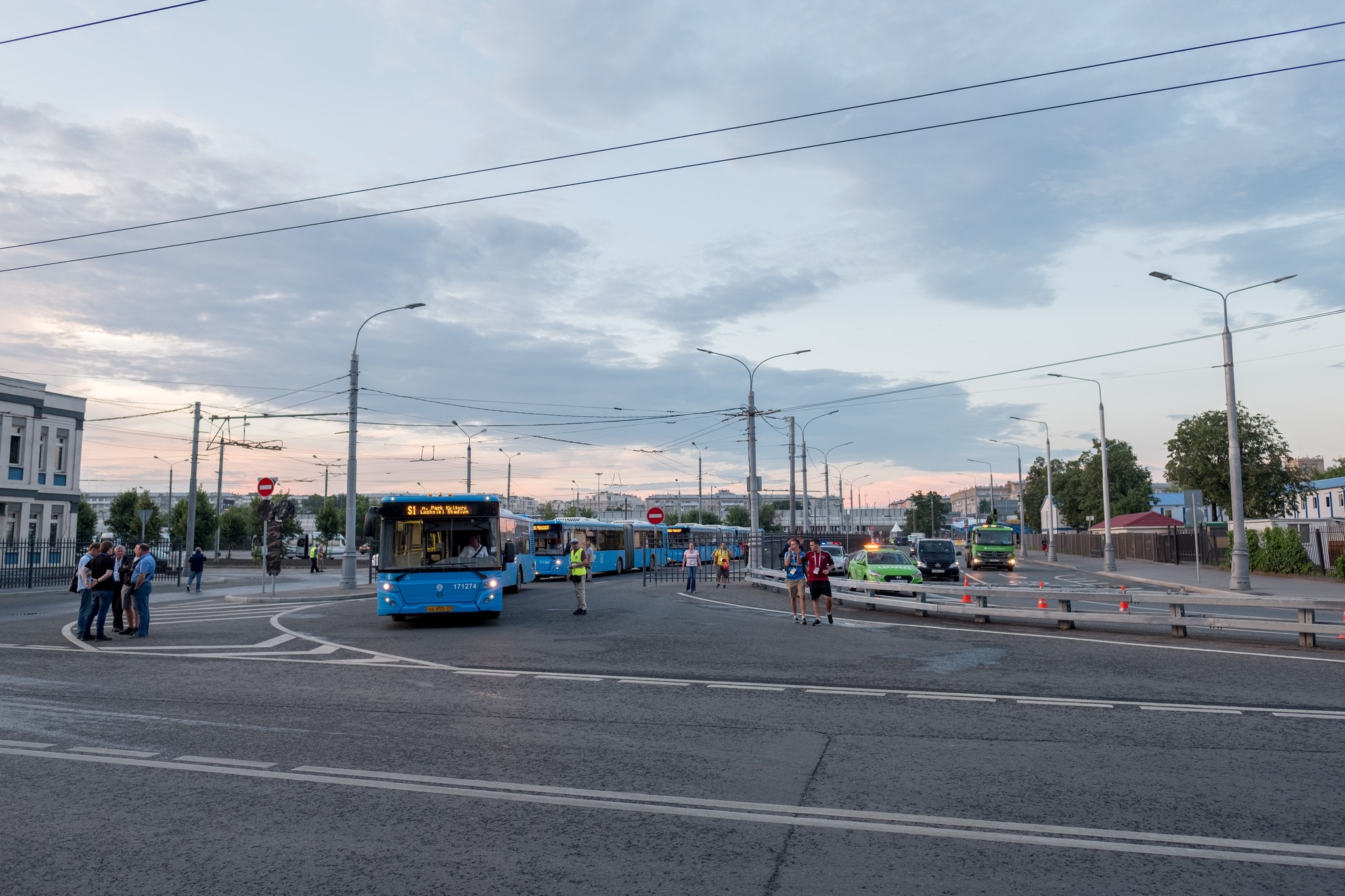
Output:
(964, 525), (970, 528)
(869, 526), (874, 530)
(257, 478), (275, 497)
(646, 507), (665, 525)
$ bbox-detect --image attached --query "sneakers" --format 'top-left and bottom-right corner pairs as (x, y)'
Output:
(812, 619), (821, 626)
(800, 617), (807, 625)
(716, 583), (721, 588)
(723, 584), (726, 589)
(573, 608), (587, 615)
(825, 612), (833, 624)
(793, 616), (799, 624)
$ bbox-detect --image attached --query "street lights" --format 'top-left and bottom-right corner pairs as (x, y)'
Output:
(864, 494), (868, 516)
(692, 442), (707, 525)
(765, 410), (840, 534)
(989, 439), (1028, 560)
(696, 347), (812, 579)
(314, 455), (341, 515)
(675, 478), (681, 524)
(843, 482), (875, 534)
(338, 302), (427, 592)
(918, 491), (944, 539)
(949, 459), (995, 527)
(887, 491), (891, 517)
(1149, 272), (1299, 592)
(818, 461), (863, 535)
(1048, 373), (1117, 571)
(833, 475), (870, 535)
(1010, 416), (1058, 562)
(901, 500), (926, 533)
(797, 442), (855, 534)
(138, 455), (248, 564)
(450, 419), (614, 521)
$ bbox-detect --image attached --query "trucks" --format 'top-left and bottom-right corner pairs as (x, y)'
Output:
(964, 509), (1020, 572)
(907, 533), (925, 544)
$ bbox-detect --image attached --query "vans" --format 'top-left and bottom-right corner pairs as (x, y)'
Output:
(893, 537), (909, 546)
(909, 538), (961, 581)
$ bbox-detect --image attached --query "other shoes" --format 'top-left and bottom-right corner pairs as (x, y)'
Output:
(316, 571), (320, 573)
(196, 588), (201, 592)
(693, 591), (696, 593)
(187, 585), (190, 591)
(312, 572), (315, 573)
(81, 634), (96, 641)
(146, 632), (148, 636)
(76, 634), (82, 639)
(113, 627), (120, 632)
(585, 580), (591, 582)
(112, 627), (124, 631)
(118, 627), (134, 635)
(685, 589), (688, 592)
(95, 634), (112, 641)
(130, 627), (139, 635)
(128, 633), (146, 638)
(320, 571), (324, 572)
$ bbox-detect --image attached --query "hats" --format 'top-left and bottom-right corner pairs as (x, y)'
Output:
(571, 538), (579, 544)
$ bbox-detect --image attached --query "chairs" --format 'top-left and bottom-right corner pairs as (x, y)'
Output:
(879, 556), (888, 564)
(869, 556), (873, 564)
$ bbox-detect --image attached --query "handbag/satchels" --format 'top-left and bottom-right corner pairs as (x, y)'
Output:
(572, 575), (582, 583)
(127, 582), (135, 596)
(67, 575), (80, 594)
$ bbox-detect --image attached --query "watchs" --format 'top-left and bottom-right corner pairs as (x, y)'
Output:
(576, 565), (577, 567)
(827, 569), (830, 573)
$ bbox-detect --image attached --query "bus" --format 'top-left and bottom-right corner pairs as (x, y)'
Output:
(271, 492), (765, 622)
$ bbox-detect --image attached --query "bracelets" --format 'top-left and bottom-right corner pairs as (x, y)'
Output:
(135, 588), (136, 589)
(97, 579), (99, 583)
(85, 580), (89, 582)
(728, 551), (730, 552)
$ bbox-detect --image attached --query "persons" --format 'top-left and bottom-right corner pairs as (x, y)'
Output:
(782, 537), (835, 627)
(310, 544), (323, 573)
(682, 542), (702, 593)
(713, 542), (733, 589)
(317, 545), (325, 572)
(566, 538), (587, 615)
(741, 538), (749, 568)
(76, 541), (156, 642)
(872, 536), (879, 545)
(187, 547), (207, 592)
(585, 543), (594, 582)
(557, 540), (566, 550)
(891, 538), (894, 545)
(459, 533), (488, 558)
(1041, 537), (1048, 556)
(956, 536), (964, 540)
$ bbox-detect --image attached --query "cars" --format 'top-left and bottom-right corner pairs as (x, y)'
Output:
(848, 546), (923, 595)
(818, 542), (849, 576)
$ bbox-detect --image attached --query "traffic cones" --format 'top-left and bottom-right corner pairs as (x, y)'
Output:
(1337, 611), (1345, 639)
(1036, 581), (1048, 608)
(960, 575), (974, 603)
(1119, 586), (1131, 615)
